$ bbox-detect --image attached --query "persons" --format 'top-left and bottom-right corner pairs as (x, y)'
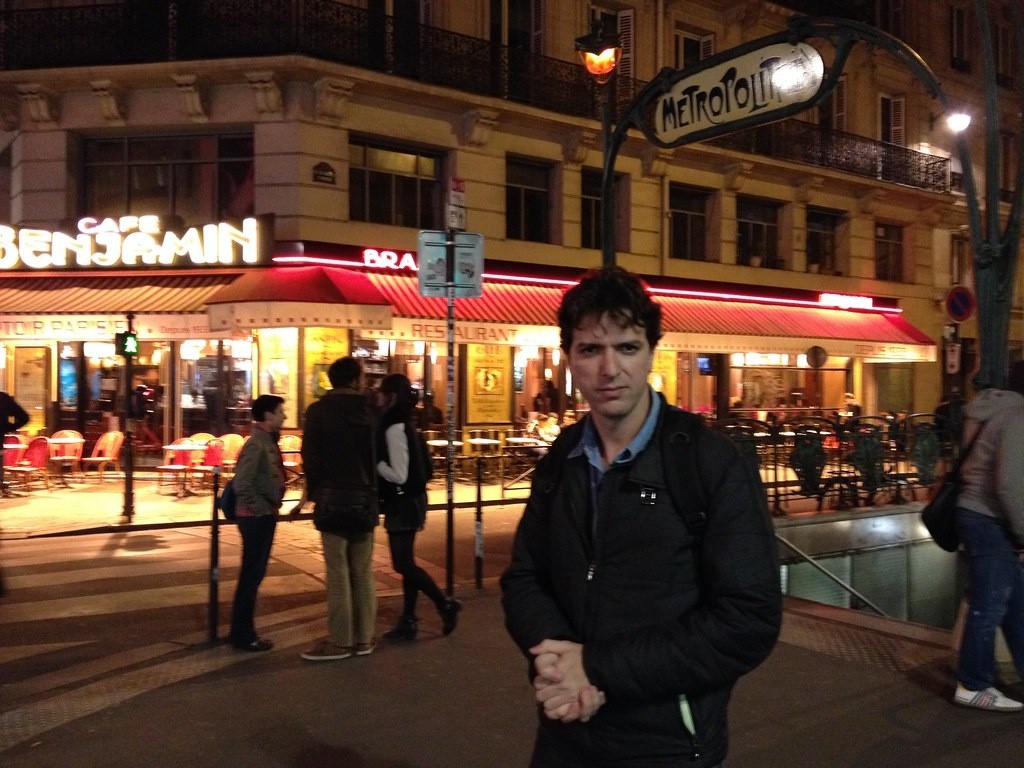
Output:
(0, 391), (31, 492)
(838, 391), (863, 428)
(126, 384), (149, 423)
(301, 354), (385, 662)
(376, 373), (462, 644)
(532, 379), (569, 415)
(955, 363), (1024, 710)
(498, 262), (783, 767)
(227, 394), (287, 654)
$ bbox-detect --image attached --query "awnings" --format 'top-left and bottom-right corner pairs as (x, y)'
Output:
(364, 270), (938, 364)
(204, 266), (394, 332)
(0, 276), (256, 341)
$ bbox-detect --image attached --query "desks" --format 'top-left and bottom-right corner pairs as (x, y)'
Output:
(48, 437), (85, 488)
(505, 437), (536, 475)
(163, 444), (208, 497)
(427, 441), (464, 487)
(468, 439), (500, 483)
(4, 444), (28, 488)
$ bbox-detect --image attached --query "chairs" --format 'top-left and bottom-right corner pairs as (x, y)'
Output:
(155, 433), (302, 488)
(1, 430), (126, 491)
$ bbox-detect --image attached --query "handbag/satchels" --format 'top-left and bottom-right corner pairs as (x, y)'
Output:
(312, 486), (380, 542)
(220, 480), (237, 521)
(921, 472), (958, 552)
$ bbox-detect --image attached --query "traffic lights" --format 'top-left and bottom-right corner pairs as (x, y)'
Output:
(136, 384), (154, 418)
(111, 332), (138, 359)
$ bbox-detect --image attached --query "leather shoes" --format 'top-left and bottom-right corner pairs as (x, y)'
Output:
(232, 639), (273, 651)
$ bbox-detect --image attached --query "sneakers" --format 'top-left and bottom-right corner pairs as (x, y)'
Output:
(356, 640), (376, 655)
(954, 681), (1024, 712)
(300, 641), (350, 659)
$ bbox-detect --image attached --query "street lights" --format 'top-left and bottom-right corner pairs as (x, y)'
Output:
(573, 13), (627, 267)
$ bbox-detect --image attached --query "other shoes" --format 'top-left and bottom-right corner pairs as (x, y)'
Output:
(438, 597), (464, 636)
(382, 617), (418, 641)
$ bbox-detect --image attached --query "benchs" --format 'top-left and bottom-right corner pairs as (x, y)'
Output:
(422, 431), (524, 475)
